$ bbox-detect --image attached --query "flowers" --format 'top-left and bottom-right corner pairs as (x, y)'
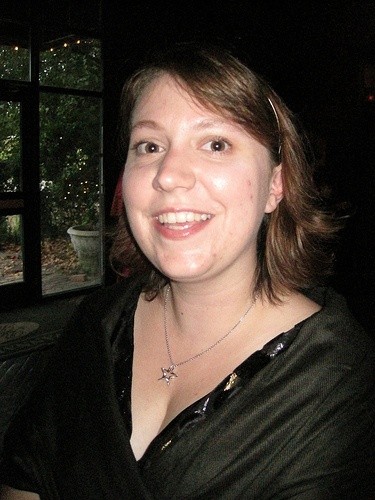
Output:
(47, 162), (98, 231)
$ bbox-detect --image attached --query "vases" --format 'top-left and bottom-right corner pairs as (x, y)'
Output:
(65, 224), (105, 280)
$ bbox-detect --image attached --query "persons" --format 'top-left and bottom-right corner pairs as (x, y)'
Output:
(1, 37), (375, 499)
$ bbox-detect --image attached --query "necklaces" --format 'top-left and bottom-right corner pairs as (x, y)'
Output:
(154, 283), (261, 387)
